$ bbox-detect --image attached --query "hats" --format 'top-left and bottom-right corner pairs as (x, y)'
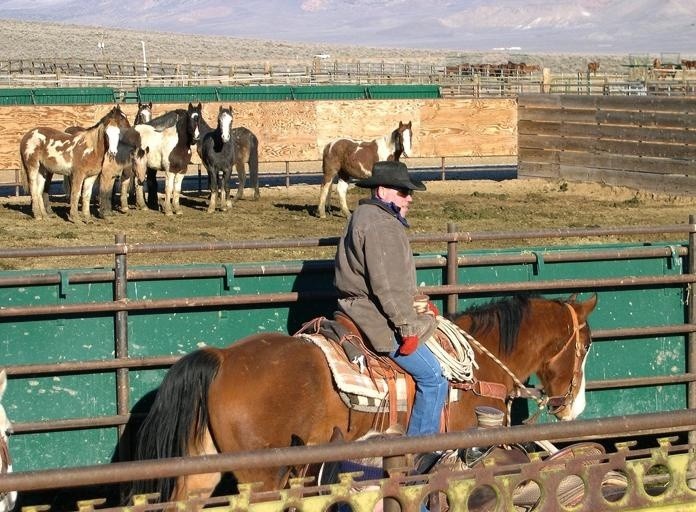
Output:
(355, 161), (426, 191)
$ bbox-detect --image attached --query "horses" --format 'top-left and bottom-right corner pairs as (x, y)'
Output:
(588, 61), (598, 75)
(653, 58), (663, 70)
(681, 59), (696, 70)
(121, 287), (599, 512)
(315, 119), (413, 220)
(17, 100), (259, 224)
(442, 60), (541, 77)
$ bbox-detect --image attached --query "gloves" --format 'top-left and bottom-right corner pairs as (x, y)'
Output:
(429, 302), (439, 316)
(400, 335), (418, 355)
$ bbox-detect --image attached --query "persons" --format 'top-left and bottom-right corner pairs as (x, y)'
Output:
(335, 428), (429, 511)
(333, 160), (448, 436)
